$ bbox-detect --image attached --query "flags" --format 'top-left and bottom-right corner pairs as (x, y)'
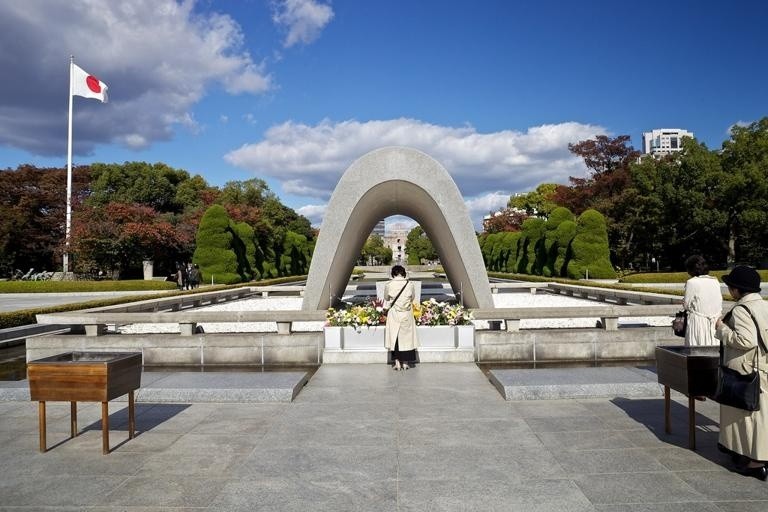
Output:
(71, 62), (109, 104)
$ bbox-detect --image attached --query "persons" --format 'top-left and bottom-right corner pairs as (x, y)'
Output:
(384, 264), (419, 371)
(174, 262), (203, 289)
(712, 264), (768, 482)
(679, 255), (724, 401)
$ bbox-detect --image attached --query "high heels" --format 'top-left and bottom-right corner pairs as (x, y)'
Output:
(717, 443), (737, 455)
(392, 365), (401, 371)
(403, 364), (409, 370)
(733, 462), (767, 481)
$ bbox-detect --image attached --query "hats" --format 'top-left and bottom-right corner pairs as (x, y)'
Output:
(721, 266), (761, 293)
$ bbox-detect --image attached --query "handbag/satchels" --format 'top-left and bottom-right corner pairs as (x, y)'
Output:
(673, 309), (688, 338)
(384, 312), (387, 316)
(714, 365), (760, 411)
